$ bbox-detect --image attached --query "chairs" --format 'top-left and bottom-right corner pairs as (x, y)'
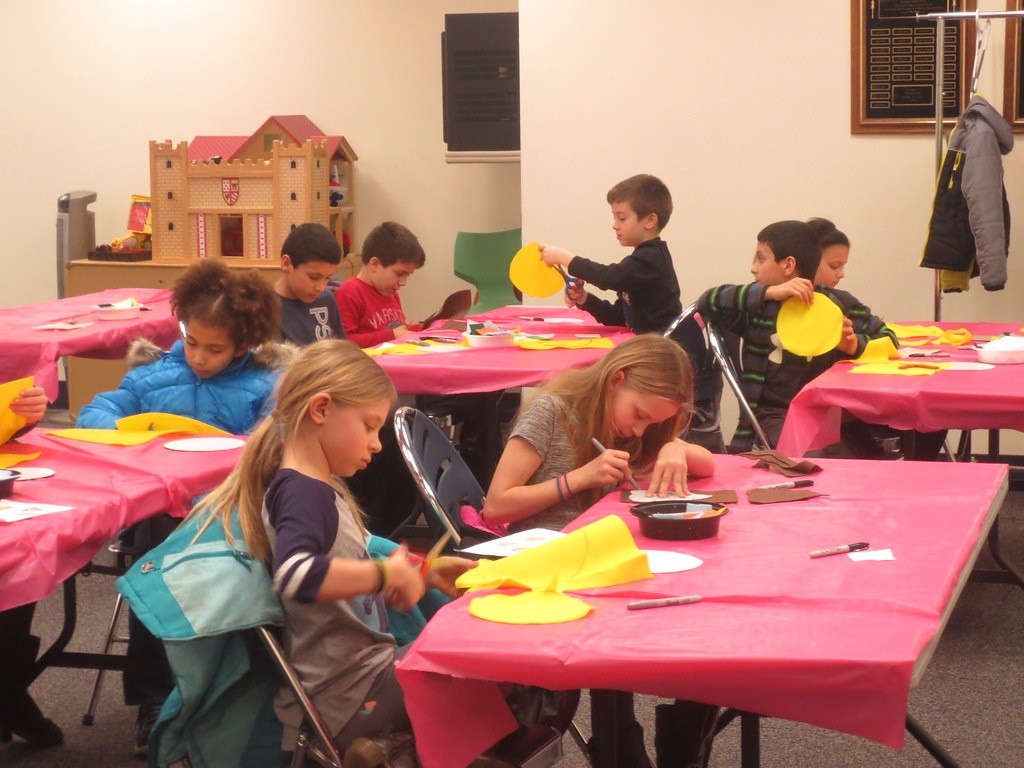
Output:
(80, 539), (144, 724)
(394, 408), (594, 763)
(113, 490), (581, 768)
(708, 321), (776, 454)
(661, 301), (725, 454)
(870, 425), (959, 466)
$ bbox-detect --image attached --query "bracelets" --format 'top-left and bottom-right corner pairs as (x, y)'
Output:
(556, 473), (573, 502)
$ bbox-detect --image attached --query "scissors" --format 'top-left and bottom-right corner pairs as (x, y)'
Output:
(896, 361), (939, 370)
(550, 263), (584, 299)
(405, 311), (438, 333)
(389, 528), (459, 610)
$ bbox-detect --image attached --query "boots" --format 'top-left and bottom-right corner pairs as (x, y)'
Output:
(588, 721), (653, 768)
(656, 704), (708, 768)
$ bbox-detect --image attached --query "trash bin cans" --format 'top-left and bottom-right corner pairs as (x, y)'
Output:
(57, 191), (97, 300)
(468, 721), (563, 768)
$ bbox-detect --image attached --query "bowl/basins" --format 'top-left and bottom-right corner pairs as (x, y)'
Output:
(0, 469), (22, 499)
(461, 330), (523, 347)
(630, 500), (728, 541)
(96, 309), (138, 320)
(971, 345), (1024, 364)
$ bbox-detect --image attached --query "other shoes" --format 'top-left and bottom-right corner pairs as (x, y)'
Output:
(0, 708), (62, 746)
(134, 718), (156, 756)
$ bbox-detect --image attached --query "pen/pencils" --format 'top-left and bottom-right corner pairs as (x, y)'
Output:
(626, 595), (704, 610)
(759, 480), (814, 489)
(908, 353), (950, 357)
(68, 317), (75, 324)
(140, 308), (152, 311)
(957, 346), (973, 349)
(518, 316), (544, 322)
(1003, 332), (1019, 337)
(420, 335), (459, 340)
(406, 339), (429, 346)
(431, 338), (458, 343)
(591, 437), (643, 491)
(810, 542), (869, 558)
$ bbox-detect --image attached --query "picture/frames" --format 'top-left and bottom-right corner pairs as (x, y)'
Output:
(850, 0), (977, 136)
(1002, 0), (1024, 134)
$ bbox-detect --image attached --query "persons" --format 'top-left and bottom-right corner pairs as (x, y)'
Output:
(538, 174), (682, 336)
(483, 332), (715, 538)
(335, 222), (424, 348)
(807, 217), (851, 289)
(0, 387), (66, 745)
(272, 223), (347, 348)
(698, 221), (900, 459)
(76, 256), (298, 754)
(224, 340), (516, 768)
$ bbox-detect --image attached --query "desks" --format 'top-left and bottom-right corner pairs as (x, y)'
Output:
(0, 425), (253, 708)
(365, 304), (641, 545)
(0, 288), (181, 404)
(395, 450), (1009, 768)
(775, 320), (1024, 592)
